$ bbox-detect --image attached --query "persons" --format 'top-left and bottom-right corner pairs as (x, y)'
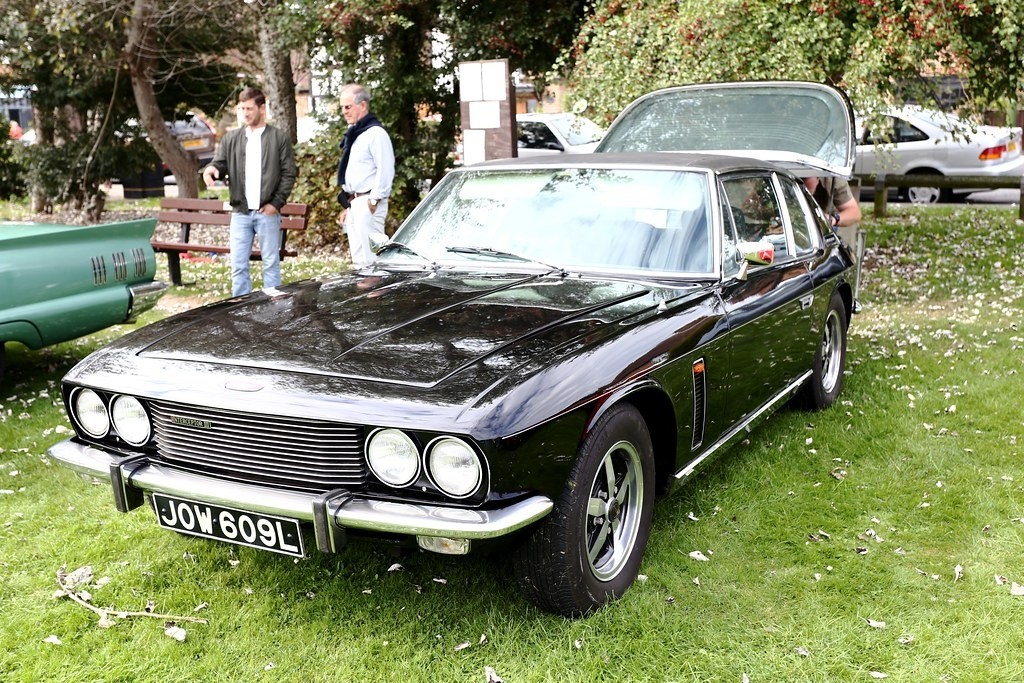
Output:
(203, 89), (297, 298)
(415, 100), (463, 186)
(8, 120), (37, 147)
(353, 269), (394, 289)
(740, 176), (863, 238)
(336, 84), (395, 269)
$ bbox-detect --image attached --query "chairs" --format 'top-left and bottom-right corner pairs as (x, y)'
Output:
(571, 201), (744, 273)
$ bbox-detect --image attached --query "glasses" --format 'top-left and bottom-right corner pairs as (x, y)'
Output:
(339, 100), (363, 111)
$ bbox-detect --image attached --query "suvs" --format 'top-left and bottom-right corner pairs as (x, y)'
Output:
(119, 109), (216, 177)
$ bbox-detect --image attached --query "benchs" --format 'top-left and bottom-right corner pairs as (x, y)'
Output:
(148, 197), (312, 287)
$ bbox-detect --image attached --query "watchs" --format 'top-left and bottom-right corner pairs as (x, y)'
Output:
(832, 211), (840, 226)
(370, 198), (379, 206)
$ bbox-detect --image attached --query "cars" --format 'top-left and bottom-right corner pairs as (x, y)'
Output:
(47, 80), (861, 622)
(851, 99), (1024, 202)
(0, 218), (168, 353)
(452, 113), (612, 164)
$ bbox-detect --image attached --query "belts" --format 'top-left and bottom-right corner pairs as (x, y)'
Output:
(347, 190), (371, 201)
